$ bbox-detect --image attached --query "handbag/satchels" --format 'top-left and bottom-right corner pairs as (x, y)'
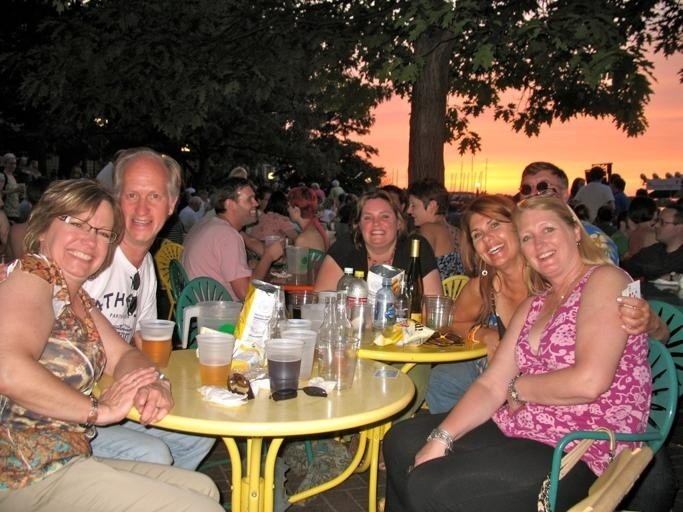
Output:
(537, 428), (654, 512)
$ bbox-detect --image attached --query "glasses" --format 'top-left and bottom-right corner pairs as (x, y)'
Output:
(269, 387), (327, 402)
(520, 181), (563, 196)
(656, 218), (676, 226)
(228, 373), (254, 400)
(57, 215), (117, 244)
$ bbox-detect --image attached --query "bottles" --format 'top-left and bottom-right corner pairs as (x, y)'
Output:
(403, 236), (423, 318)
(347, 270), (368, 342)
(335, 268), (354, 293)
(319, 296), (339, 382)
(372, 278), (396, 330)
(335, 290), (351, 377)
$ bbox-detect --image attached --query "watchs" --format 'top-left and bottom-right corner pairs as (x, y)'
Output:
(427, 427), (456, 451)
(508, 375), (527, 405)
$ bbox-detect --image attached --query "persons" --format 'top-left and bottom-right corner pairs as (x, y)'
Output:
(317, 198), (338, 227)
(383, 185), (408, 214)
(407, 179), (476, 280)
(250, 191), (298, 242)
(14, 153), (32, 186)
(446, 200), (468, 228)
(96, 149), (128, 188)
(636, 189), (648, 198)
(592, 205), (616, 237)
(310, 183), (326, 204)
(627, 195), (660, 258)
(0, 153), (28, 225)
(229, 167), (248, 180)
(338, 193), (346, 207)
(345, 193), (357, 206)
(426, 193), (670, 413)
(179, 196), (202, 234)
(335, 205), (359, 238)
(184, 187), (196, 201)
(81, 148), (180, 353)
(382, 194), (652, 512)
(179, 177), (287, 304)
(68, 157), (84, 179)
(0, 177), (225, 512)
(297, 182), (306, 188)
(316, 190), (444, 298)
(198, 189), (211, 209)
(329, 180), (344, 209)
(574, 204), (590, 221)
(518, 162), (619, 267)
(569, 166), (615, 221)
(620, 207), (683, 306)
(614, 180), (630, 212)
(611, 174), (620, 184)
(246, 185), (272, 235)
(279, 187), (328, 276)
(610, 210), (639, 261)
(568, 178), (585, 202)
(29, 158), (41, 179)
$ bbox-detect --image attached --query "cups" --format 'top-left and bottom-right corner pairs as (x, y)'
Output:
(286, 245), (309, 286)
(197, 334), (235, 390)
(321, 336), (359, 389)
(301, 304), (325, 333)
(264, 236), (279, 249)
(199, 301), (242, 335)
(349, 306), (373, 347)
(264, 339), (304, 401)
(290, 291), (316, 310)
(140, 320), (175, 368)
(17, 183), (25, 191)
(281, 330), (317, 381)
(425, 295), (453, 338)
(278, 319), (311, 336)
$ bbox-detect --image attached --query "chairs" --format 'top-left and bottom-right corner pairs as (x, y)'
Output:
(642, 300), (683, 398)
(157, 243), (185, 320)
(176, 276), (233, 350)
(170, 259), (190, 302)
(441, 275), (470, 303)
(545, 338), (678, 512)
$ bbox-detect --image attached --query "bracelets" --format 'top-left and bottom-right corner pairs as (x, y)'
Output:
(85, 393), (98, 428)
(467, 323), (480, 344)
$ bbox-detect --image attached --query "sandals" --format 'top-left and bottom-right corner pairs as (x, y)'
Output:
(349, 437), (368, 463)
(378, 440), (387, 473)
